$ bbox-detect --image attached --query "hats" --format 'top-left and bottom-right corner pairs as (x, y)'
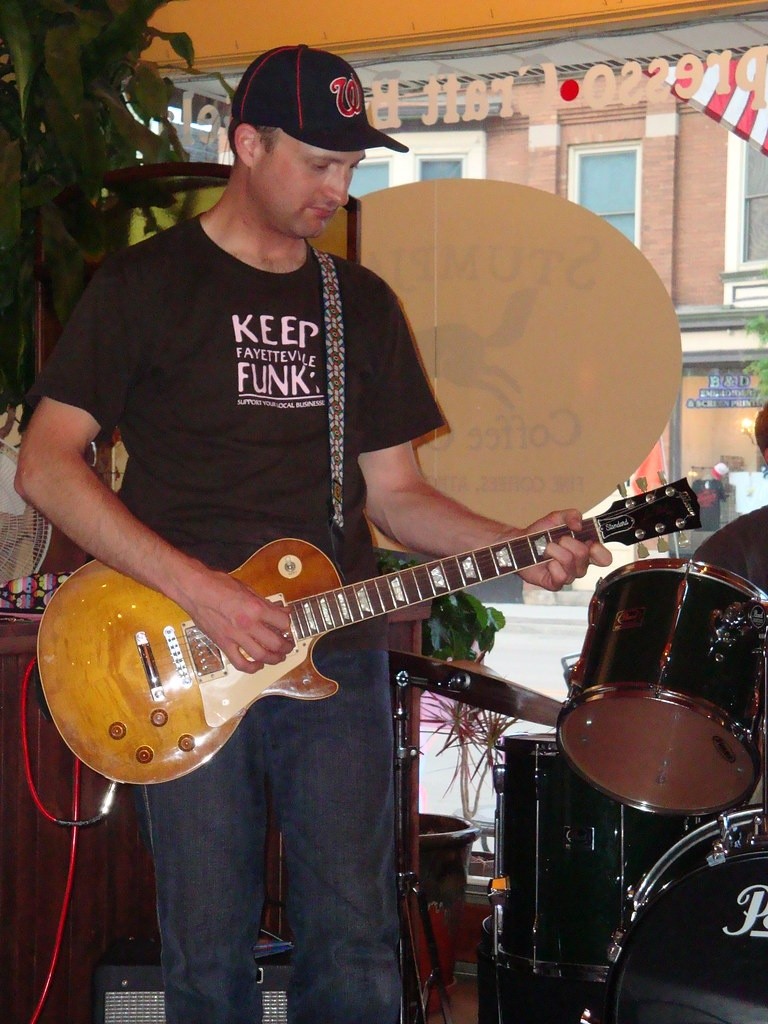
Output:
(233, 44), (409, 153)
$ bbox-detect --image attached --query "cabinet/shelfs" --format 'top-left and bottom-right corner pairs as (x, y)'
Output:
(0, 597), (435, 1024)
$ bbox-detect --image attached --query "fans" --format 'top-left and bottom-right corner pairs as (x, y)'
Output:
(0, 437), (51, 584)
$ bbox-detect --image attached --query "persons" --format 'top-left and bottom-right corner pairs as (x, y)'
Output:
(692, 476), (726, 531)
(13, 43), (614, 1024)
(693, 403), (768, 597)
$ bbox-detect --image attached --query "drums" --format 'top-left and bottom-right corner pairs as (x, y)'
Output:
(556, 556), (767, 816)
(483, 730), (730, 984)
(595, 801), (768, 1024)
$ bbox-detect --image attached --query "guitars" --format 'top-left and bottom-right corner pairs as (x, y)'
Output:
(36, 470), (704, 789)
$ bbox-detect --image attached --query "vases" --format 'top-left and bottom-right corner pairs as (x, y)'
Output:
(419, 811), (482, 905)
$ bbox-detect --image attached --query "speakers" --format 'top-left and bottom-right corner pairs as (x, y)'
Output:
(91, 957), (289, 1024)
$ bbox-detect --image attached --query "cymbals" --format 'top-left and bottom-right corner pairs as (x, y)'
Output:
(386, 647), (563, 730)
